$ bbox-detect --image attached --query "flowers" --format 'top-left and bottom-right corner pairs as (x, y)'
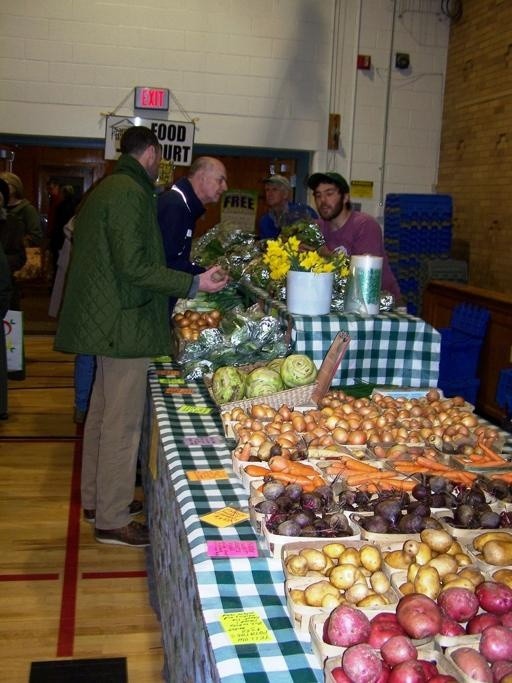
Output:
(262, 235), (350, 280)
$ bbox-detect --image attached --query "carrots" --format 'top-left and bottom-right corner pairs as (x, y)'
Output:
(390, 450), (478, 486)
(462, 442), (510, 468)
(490, 472), (512, 484)
(244, 455), (324, 491)
(318, 454), (419, 492)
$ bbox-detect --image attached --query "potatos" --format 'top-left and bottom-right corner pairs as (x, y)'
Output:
(385, 529), (484, 598)
(175, 310), (222, 339)
(288, 541), (390, 612)
(474, 531), (512, 590)
(326, 580), (512, 683)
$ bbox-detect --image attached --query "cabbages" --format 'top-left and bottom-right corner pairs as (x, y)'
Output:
(246, 367), (284, 398)
(266, 357), (285, 373)
(280, 354), (318, 387)
(212, 366), (246, 403)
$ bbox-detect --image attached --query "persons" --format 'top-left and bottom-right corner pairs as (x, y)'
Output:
(47, 219), (75, 319)
(1, 172), (41, 381)
(305, 172), (403, 310)
(256, 174), (318, 242)
(0, 178), (28, 422)
(49, 215), (95, 424)
(134, 156), (227, 487)
(52, 126), (229, 549)
(47, 177), (76, 295)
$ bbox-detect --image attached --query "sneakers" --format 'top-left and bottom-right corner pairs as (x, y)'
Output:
(93, 521), (152, 548)
(83, 497), (146, 526)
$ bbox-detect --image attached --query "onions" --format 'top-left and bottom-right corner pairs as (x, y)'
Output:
(226, 389), (499, 457)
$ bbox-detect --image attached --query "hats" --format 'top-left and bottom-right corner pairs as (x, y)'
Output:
(305, 171), (350, 194)
(260, 173), (297, 194)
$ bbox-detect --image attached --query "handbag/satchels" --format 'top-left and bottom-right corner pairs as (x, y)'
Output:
(13, 241), (44, 285)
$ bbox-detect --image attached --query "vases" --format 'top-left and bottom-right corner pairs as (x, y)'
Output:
(286, 270), (333, 315)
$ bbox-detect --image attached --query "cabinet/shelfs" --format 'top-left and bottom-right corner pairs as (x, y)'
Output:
(139, 259), (512, 627)
